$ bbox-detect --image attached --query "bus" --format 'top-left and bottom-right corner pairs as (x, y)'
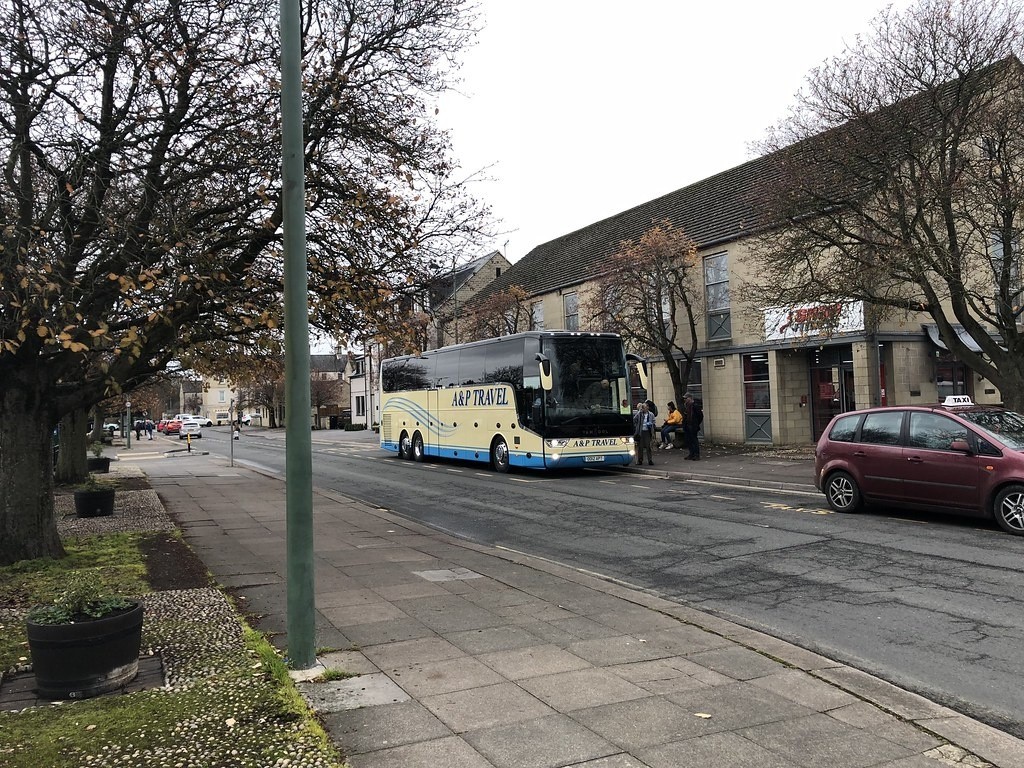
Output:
(379, 329), (649, 473)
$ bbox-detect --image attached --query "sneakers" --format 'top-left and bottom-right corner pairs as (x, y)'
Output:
(665, 444), (674, 450)
(659, 443), (666, 450)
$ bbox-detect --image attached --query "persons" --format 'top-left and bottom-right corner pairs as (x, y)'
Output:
(659, 401), (683, 450)
(234, 420), (241, 440)
(146, 420), (153, 440)
(135, 422), (141, 440)
(682, 393), (703, 460)
(633, 403), (656, 466)
(584, 379), (609, 402)
(535, 389), (559, 406)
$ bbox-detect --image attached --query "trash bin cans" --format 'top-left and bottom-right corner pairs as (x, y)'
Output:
(330, 414), (338, 429)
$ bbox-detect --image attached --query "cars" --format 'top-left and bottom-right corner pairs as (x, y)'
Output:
(242, 413), (262, 426)
(179, 422), (202, 440)
(143, 420), (156, 430)
(157, 420), (169, 432)
(167, 421), (183, 433)
(813, 395), (1024, 537)
(103, 422), (119, 431)
(175, 414), (213, 427)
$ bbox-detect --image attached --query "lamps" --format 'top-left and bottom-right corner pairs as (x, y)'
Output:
(799, 395), (807, 407)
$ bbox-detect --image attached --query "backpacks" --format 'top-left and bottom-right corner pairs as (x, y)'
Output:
(645, 400), (658, 417)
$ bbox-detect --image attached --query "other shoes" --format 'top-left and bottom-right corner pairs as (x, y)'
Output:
(684, 454), (692, 460)
(693, 456), (701, 461)
(637, 461), (642, 466)
(648, 460), (654, 466)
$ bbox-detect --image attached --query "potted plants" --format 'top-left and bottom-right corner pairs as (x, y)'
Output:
(74, 473), (115, 517)
(86, 441), (110, 474)
(26, 570), (145, 698)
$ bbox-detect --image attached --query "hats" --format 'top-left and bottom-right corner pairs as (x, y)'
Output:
(682, 392), (693, 398)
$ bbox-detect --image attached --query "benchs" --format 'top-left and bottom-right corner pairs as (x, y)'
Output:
(656, 427), (684, 449)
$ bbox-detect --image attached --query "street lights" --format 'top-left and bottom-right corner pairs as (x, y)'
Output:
(429, 256), (458, 345)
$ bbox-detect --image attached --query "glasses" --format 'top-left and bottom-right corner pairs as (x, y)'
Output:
(683, 398), (689, 400)
